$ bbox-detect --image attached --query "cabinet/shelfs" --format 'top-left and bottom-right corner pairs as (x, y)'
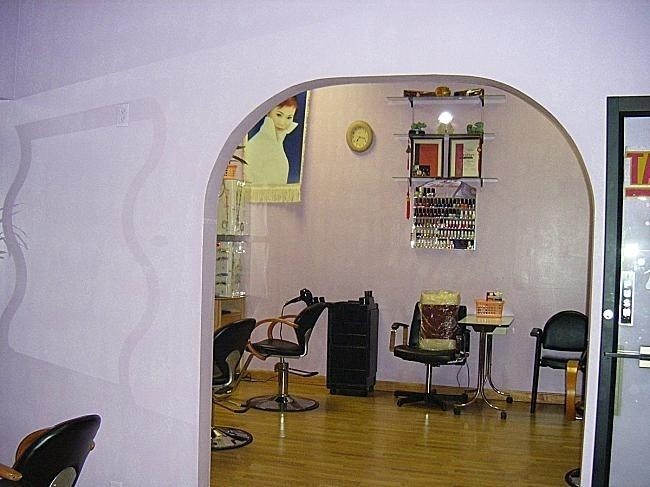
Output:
(215, 295), (245, 328)
(324, 296), (378, 398)
(384, 91), (505, 183)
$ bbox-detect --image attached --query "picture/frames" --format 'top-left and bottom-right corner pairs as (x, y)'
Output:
(448, 135), (483, 179)
(410, 138), (445, 178)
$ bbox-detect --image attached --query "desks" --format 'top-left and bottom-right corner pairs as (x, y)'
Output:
(450, 313), (518, 420)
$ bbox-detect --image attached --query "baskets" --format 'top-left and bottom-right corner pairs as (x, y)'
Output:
(476, 298), (504, 317)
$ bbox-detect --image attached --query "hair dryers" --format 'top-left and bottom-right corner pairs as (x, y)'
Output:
(284, 288), (315, 308)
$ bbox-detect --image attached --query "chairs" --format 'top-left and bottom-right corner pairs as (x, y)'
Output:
(531, 309), (590, 419)
(385, 296), (474, 414)
(245, 290), (331, 412)
(1, 414), (101, 487)
(208, 316), (257, 449)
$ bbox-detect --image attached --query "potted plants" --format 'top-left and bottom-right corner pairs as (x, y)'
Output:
(223, 144), (248, 180)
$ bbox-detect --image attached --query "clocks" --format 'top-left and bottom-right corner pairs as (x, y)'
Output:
(344, 120), (374, 152)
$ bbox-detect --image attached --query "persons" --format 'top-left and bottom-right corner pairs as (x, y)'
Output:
(247, 96), (299, 185)
